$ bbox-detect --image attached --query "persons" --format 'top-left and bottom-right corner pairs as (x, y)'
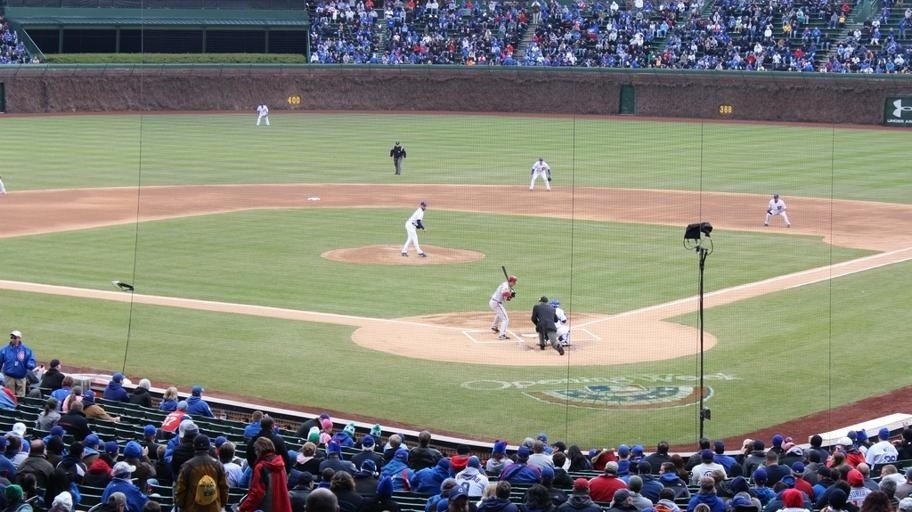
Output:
(0, 179), (5, 193)
(401, 201), (428, 257)
(764, 194), (791, 228)
(536, 299), (570, 347)
(488, 275), (517, 339)
(531, 296), (564, 355)
(0, 15), (40, 64)
(256, 103), (270, 126)
(390, 141), (406, 176)
(529, 157), (552, 191)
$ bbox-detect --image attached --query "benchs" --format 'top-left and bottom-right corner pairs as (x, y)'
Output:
(304, 1), (912, 74)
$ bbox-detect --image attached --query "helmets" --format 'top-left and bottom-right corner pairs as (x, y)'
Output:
(420, 200), (427, 209)
(508, 275), (517, 283)
(538, 296), (549, 303)
(549, 300), (560, 308)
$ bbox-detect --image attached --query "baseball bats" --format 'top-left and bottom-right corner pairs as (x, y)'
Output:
(502, 266), (515, 297)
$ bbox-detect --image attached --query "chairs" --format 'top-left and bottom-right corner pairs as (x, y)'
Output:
(869, 459), (910, 482)
(486, 467), (810, 512)
(1, 377), (483, 512)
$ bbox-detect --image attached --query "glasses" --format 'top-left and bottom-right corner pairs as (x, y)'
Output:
(10, 336), (18, 339)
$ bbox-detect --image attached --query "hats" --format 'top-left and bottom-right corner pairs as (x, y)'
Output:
(467, 426), (912, 512)
(10, 330), (22, 338)
(291, 413), (468, 503)
(0, 360), (288, 512)
(396, 141), (400, 145)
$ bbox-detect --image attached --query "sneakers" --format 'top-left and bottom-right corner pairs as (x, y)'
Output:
(418, 252), (427, 257)
(491, 328), (499, 334)
(401, 253), (409, 257)
